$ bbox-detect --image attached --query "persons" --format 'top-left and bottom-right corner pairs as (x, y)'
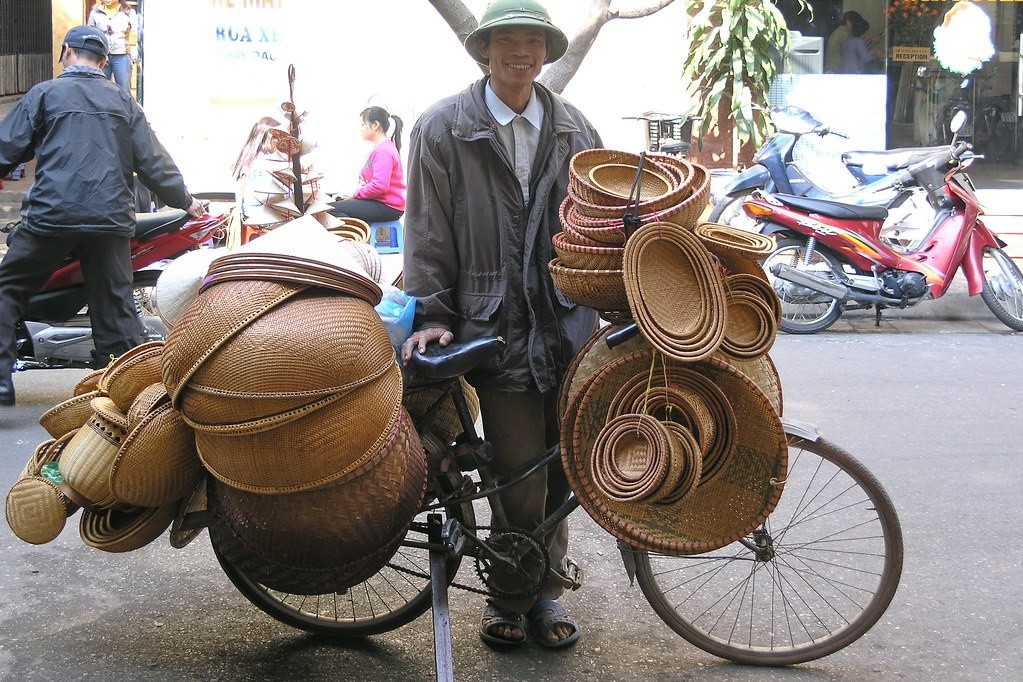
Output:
(322, 105), (407, 227)
(84, 0), (137, 97)
(399, 0), (613, 648)
(840, 20), (882, 75)
(0, 25), (208, 405)
(232, 117), (300, 231)
(826, 11), (882, 75)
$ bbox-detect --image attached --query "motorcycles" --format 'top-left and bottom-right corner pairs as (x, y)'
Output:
(920, 66), (1020, 159)
(742, 108), (1023, 334)
(706, 101), (975, 255)
(0, 163), (231, 373)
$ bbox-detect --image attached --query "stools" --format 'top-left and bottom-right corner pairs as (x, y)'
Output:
(241, 225), (260, 245)
(369, 220), (404, 255)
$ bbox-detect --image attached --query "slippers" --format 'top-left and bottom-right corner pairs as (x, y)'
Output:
(526, 600), (580, 649)
(479, 606), (527, 646)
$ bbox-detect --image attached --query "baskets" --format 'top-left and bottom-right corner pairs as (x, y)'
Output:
(4, 214), (429, 596)
(546, 148), (791, 557)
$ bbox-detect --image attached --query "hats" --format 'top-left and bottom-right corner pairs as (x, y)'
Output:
(58, 25), (110, 64)
(464, 0), (569, 68)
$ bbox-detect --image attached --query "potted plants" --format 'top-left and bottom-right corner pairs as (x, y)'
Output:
(680, 0), (815, 197)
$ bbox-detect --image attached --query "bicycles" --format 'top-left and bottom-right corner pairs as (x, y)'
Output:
(624, 110), (705, 158)
(182, 314), (905, 669)
